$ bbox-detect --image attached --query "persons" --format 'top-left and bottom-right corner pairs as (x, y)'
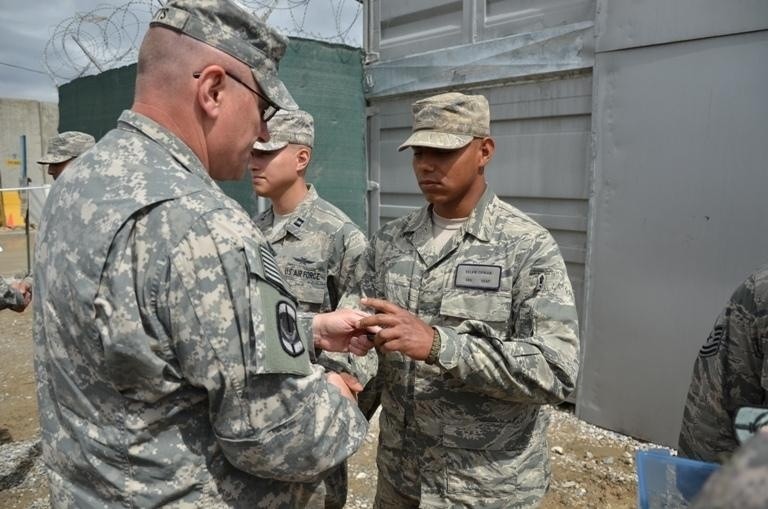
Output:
(678, 430), (768, 507)
(674, 264), (768, 496)
(10, 129), (96, 310)
(248, 102), (374, 509)
(0, 248), (31, 314)
(360, 89), (588, 508)
(32, 1), (383, 509)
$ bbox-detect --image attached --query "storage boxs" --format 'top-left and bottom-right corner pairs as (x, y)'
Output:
(637, 449), (721, 508)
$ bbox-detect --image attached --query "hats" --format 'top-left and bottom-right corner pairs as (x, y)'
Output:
(36, 131), (95, 165)
(398, 92), (490, 152)
(149, 0), (314, 152)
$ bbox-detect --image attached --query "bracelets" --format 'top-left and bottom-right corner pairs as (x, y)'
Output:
(424, 328), (441, 366)
